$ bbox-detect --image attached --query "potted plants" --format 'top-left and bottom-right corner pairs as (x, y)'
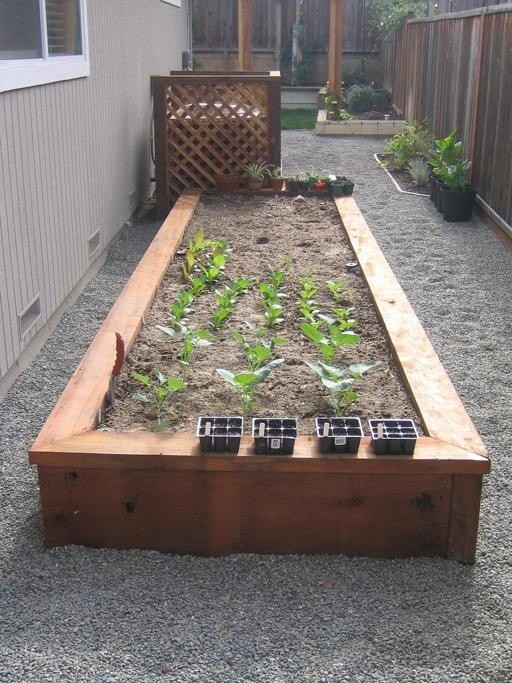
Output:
(431, 135), (478, 222)
(241, 160), (351, 197)
